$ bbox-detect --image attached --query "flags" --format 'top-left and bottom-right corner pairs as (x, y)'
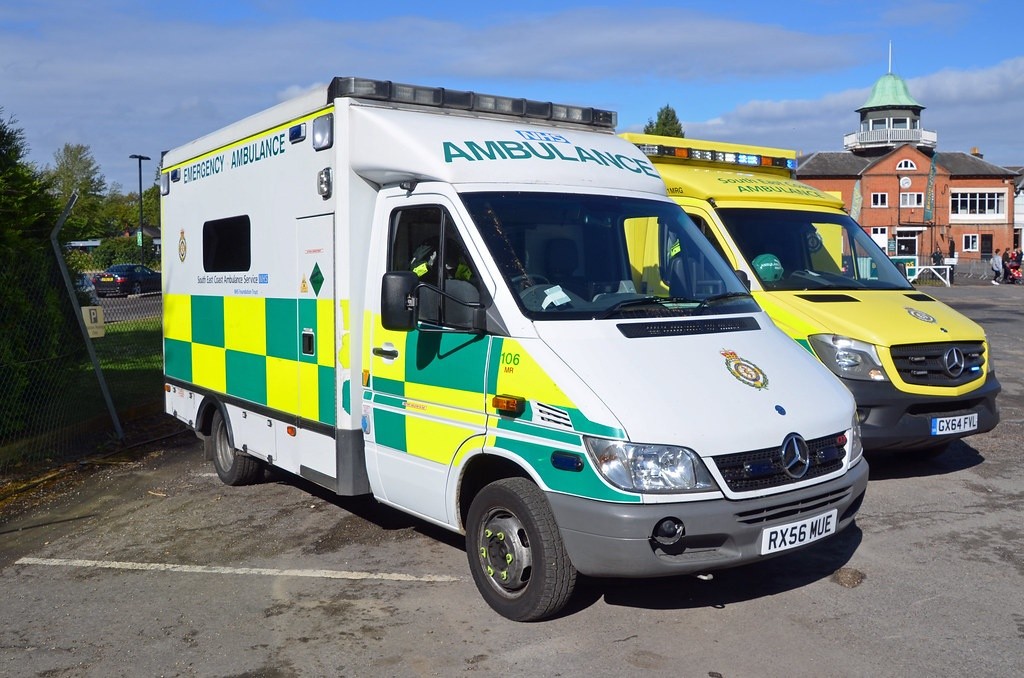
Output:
(850, 179), (864, 224)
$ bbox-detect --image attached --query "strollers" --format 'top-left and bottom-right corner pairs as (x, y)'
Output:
(1007, 261), (1024, 285)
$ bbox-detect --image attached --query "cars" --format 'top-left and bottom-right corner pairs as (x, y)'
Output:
(72, 272), (100, 306)
(92, 263), (161, 299)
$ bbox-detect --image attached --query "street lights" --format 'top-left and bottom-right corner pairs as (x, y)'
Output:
(129, 154), (151, 266)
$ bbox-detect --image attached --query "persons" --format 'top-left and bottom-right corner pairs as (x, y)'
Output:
(930, 246), (943, 279)
(1000, 245), (1024, 285)
(991, 249), (1002, 286)
(948, 236), (955, 258)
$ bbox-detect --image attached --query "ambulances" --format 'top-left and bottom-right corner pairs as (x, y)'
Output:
(619, 134), (1002, 458)
(161, 75), (869, 623)
